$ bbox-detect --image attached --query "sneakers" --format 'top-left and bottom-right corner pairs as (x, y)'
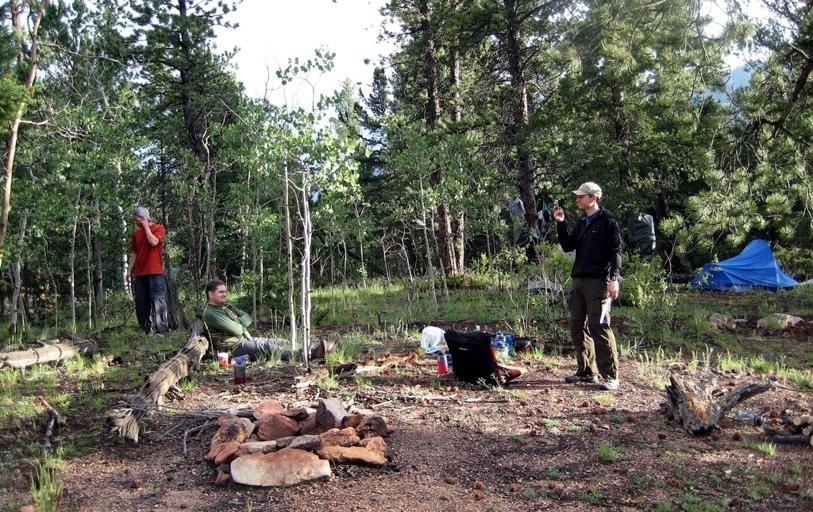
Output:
(565, 373), (619, 390)
(313, 334), (341, 358)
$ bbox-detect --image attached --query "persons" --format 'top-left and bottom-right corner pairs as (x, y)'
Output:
(203, 280), (341, 361)
(553, 182), (622, 390)
(126, 207), (168, 335)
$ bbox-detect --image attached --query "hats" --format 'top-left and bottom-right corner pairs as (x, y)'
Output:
(134, 206), (150, 220)
(571, 182), (602, 199)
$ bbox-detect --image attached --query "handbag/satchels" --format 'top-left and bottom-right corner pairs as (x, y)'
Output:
(443, 327), (521, 385)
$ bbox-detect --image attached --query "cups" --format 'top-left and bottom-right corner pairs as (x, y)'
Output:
(218, 353), (228, 369)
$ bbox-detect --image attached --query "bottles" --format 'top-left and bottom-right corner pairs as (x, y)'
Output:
(505, 335), (512, 356)
(438, 351), (448, 373)
(235, 358), (246, 385)
(491, 331), (506, 348)
(734, 409), (764, 427)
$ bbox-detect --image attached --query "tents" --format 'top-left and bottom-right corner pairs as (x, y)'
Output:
(690, 239), (799, 291)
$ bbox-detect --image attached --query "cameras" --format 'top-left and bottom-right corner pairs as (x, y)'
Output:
(554, 199), (559, 210)
